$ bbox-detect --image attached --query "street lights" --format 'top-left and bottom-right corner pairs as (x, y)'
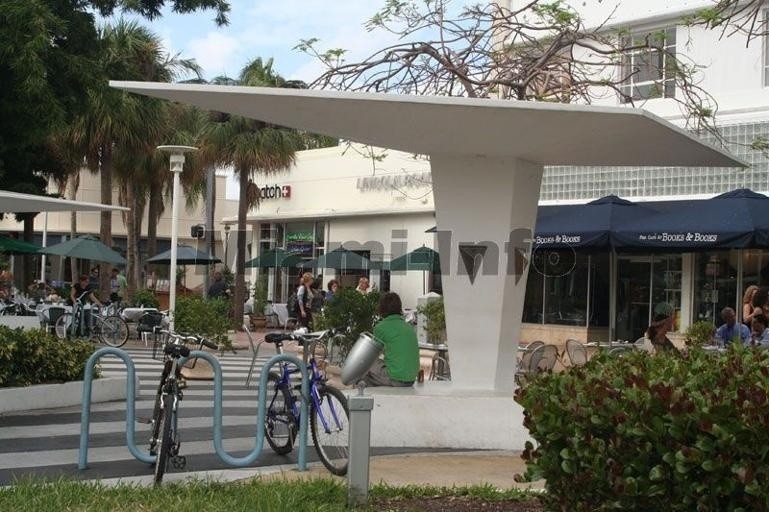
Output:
(156, 142), (200, 346)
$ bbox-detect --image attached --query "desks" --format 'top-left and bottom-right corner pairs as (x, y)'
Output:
(585, 338), (642, 365)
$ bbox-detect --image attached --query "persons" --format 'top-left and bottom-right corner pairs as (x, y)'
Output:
(645, 314), (684, 359)
(352, 292), (419, 387)
(288, 271), (369, 332)
(0, 264), (103, 316)
(714, 283), (769, 347)
(207, 268), (234, 300)
(108, 269), (130, 310)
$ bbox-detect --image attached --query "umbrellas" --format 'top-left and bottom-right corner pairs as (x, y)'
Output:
(35, 233), (130, 266)
(1, 235), (42, 254)
(245, 242), (442, 295)
(146, 243), (221, 300)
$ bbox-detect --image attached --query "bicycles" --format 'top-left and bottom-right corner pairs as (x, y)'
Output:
(262, 331), (355, 475)
(55, 295), (130, 347)
(135, 324), (219, 490)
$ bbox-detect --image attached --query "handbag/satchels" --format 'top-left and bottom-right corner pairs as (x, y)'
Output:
(139, 311), (165, 326)
(65, 297), (86, 307)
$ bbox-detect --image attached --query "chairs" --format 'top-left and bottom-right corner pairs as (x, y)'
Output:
(40, 307), (99, 341)
(138, 310), (163, 347)
(515, 333), (588, 380)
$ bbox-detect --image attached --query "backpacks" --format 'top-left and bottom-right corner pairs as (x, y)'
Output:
(286, 283), (307, 318)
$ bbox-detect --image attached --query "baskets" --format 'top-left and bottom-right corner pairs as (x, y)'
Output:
(152, 332), (201, 368)
